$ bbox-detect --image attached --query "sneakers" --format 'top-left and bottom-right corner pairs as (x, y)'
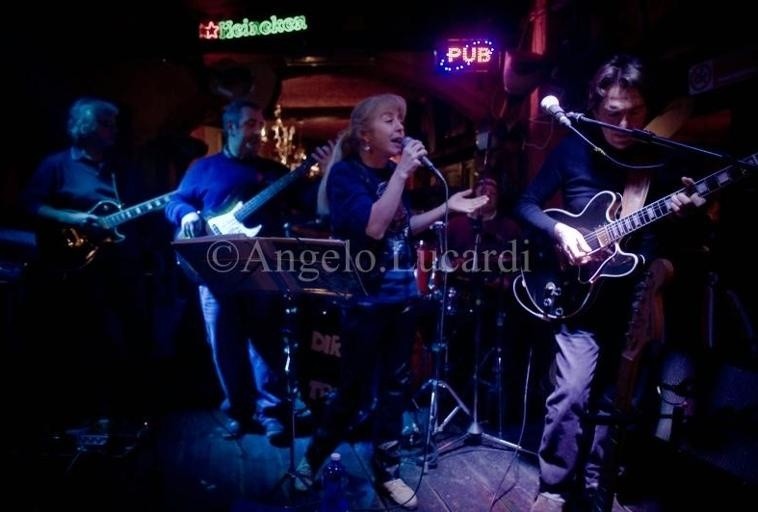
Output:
(531, 496), (565, 511)
(372, 474), (417, 507)
(260, 415), (290, 440)
(221, 417), (254, 437)
(296, 457), (315, 490)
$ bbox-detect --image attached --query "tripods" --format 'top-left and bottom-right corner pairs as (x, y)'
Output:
(417, 231), (539, 470)
(263, 292), (324, 512)
(407, 186), (488, 474)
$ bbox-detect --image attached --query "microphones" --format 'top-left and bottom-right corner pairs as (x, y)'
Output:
(540, 95), (571, 126)
(403, 137), (435, 171)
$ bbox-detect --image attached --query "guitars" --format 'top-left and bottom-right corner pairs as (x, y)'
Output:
(593, 257), (675, 511)
(520, 153), (758, 322)
(43, 190), (177, 279)
(174, 140), (335, 286)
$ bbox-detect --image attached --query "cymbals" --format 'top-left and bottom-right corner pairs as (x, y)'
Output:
(480, 255), (521, 263)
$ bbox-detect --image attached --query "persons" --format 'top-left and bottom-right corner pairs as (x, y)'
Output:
(163, 97), (337, 446)
(516, 57), (711, 512)
(319, 94), (490, 510)
(16, 92), (167, 434)
